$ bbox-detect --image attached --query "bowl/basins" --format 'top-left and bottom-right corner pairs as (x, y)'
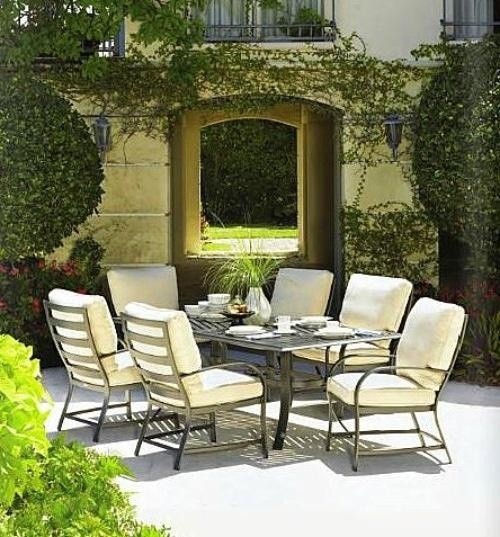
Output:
(184, 293), (231, 323)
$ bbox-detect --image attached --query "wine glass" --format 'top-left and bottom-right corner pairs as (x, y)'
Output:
(220, 306), (256, 325)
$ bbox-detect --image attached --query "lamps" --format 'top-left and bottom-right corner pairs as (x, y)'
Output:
(92, 111), (113, 152)
(383, 113), (403, 162)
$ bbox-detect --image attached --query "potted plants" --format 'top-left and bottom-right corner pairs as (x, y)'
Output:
(199, 205), (284, 325)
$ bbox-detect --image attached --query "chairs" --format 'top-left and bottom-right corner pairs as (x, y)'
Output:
(325, 295), (470, 473)
(106, 264), (211, 344)
(42, 288), (179, 442)
(120, 301), (268, 471)
(289, 273), (413, 420)
(270, 268), (333, 380)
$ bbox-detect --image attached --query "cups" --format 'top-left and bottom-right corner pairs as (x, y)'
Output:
(275, 315), (291, 331)
(327, 320), (339, 328)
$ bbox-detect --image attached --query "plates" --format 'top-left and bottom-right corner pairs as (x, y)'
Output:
(273, 329), (297, 335)
(313, 328), (354, 340)
(298, 315), (333, 328)
(224, 324), (266, 337)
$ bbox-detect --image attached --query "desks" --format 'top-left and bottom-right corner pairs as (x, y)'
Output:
(110, 316), (401, 450)
